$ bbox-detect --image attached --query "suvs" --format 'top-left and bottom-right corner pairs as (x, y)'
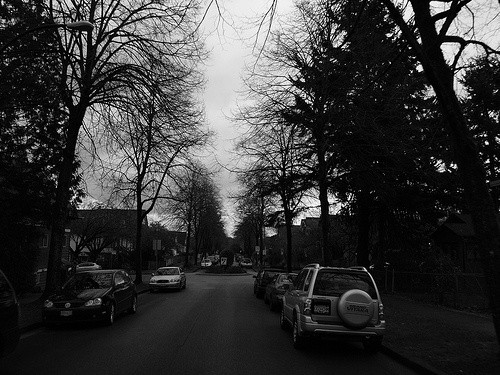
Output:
(280, 263), (387, 355)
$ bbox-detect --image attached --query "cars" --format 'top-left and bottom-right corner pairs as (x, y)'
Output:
(200, 254), (220, 268)
(265, 272), (298, 312)
(68, 262), (102, 275)
(0, 271), (21, 348)
(149, 266), (186, 293)
(253, 268), (284, 299)
(235, 254), (253, 268)
(41, 269), (137, 326)
